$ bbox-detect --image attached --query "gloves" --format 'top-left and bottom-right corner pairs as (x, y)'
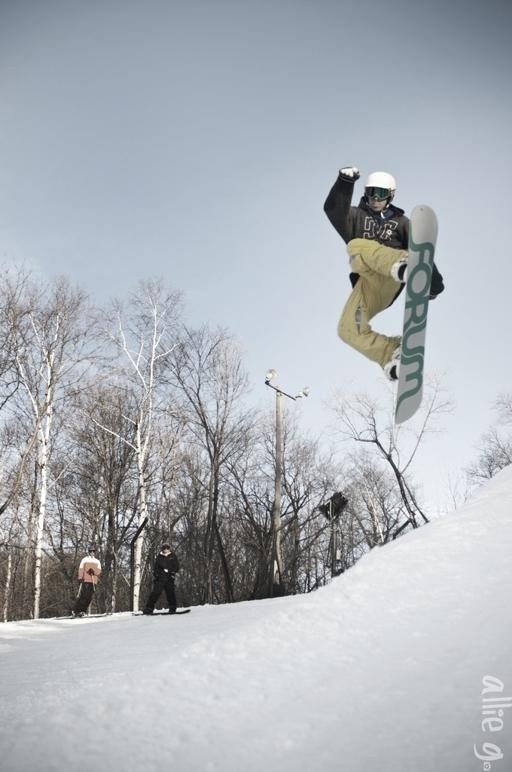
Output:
(338, 166), (361, 183)
(364, 171), (396, 202)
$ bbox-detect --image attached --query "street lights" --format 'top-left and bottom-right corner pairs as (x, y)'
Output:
(265, 364), (311, 596)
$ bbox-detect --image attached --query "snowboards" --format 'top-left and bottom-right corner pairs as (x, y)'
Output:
(134, 610), (190, 615)
(395, 204), (438, 425)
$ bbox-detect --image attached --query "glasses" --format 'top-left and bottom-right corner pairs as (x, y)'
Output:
(367, 187), (393, 201)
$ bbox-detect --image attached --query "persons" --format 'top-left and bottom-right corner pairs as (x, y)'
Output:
(142, 542), (180, 614)
(322, 166), (445, 382)
(72, 545), (103, 618)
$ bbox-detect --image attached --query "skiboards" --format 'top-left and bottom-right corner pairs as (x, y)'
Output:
(54, 613), (112, 619)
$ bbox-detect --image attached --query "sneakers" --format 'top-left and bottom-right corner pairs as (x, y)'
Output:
(383, 344), (402, 382)
(390, 257), (408, 284)
